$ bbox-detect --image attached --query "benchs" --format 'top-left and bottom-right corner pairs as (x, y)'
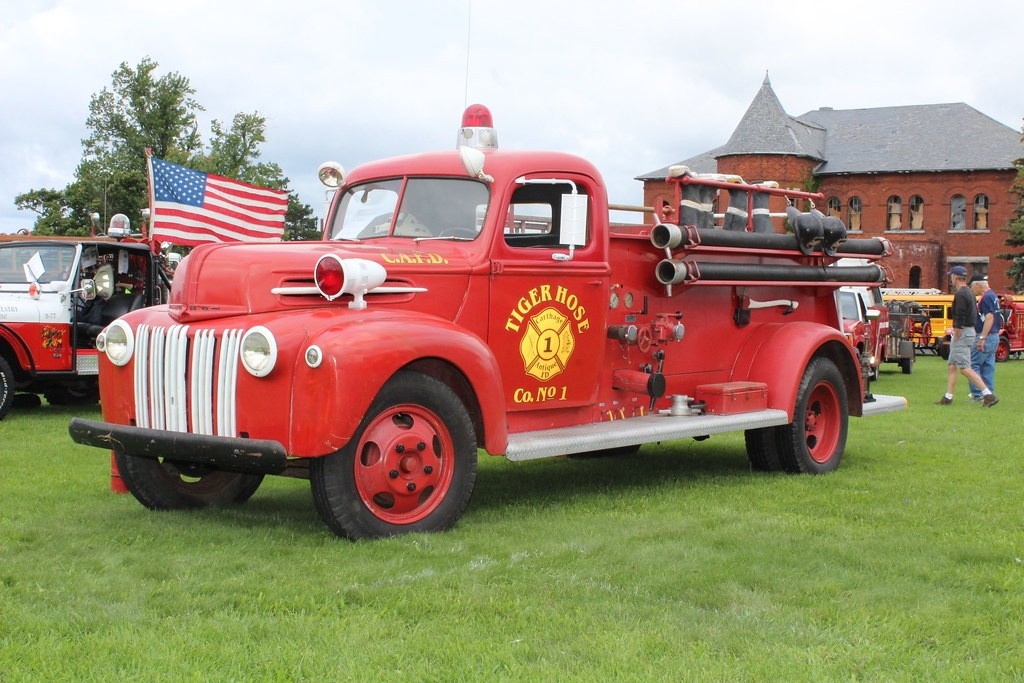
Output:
(908, 336), (940, 355)
(504, 235), (557, 248)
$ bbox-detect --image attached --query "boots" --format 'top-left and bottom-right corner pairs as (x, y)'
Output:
(723, 175), (780, 234)
(668, 164), (727, 229)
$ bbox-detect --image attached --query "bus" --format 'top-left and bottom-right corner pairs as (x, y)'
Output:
(883, 295), (1024, 344)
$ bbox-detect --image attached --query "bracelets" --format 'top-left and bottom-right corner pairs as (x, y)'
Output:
(979, 336), (985, 339)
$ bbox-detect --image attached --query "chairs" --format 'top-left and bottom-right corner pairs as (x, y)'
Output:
(70, 290), (143, 349)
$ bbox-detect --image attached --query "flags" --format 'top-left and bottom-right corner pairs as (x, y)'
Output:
(147, 155), (290, 252)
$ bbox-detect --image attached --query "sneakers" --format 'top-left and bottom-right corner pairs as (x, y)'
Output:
(982, 394), (999, 407)
(970, 396), (984, 402)
(934, 395), (953, 405)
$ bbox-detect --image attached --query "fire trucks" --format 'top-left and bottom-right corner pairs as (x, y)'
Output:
(68, 105), (906, 542)
(833, 285), (913, 381)
(0, 208), (181, 421)
(940, 295), (1024, 362)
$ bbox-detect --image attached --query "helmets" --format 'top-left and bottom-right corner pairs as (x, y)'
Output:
(786, 205), (824, 255)
(810, 209), (847, 256)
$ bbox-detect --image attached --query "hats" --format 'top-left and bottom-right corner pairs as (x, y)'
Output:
(966, 273), (989, 285)
(946, 266), (968, 277)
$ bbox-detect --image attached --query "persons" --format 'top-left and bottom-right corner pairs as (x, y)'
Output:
(921, 311), (930, 328)
(967, 273), (1003, 402)
(934, 266), (998, 408)
